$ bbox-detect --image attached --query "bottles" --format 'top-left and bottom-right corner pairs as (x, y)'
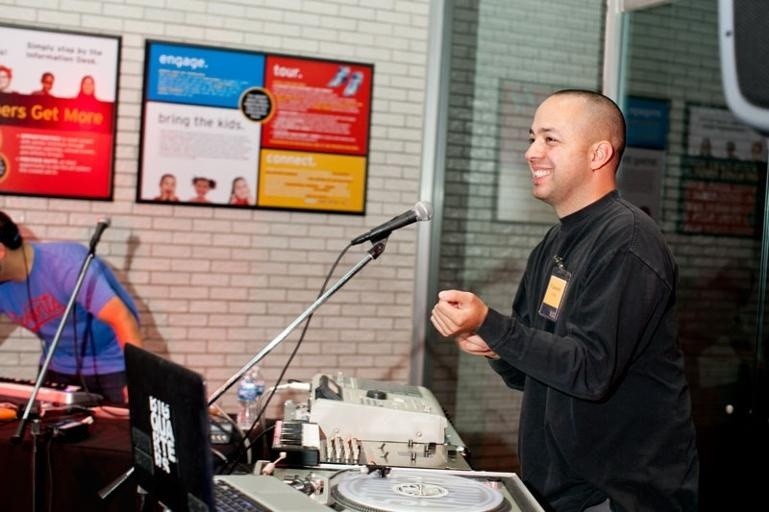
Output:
(238, 360), (265, 430)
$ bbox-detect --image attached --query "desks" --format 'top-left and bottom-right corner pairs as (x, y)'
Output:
(1, 405), (139, 510)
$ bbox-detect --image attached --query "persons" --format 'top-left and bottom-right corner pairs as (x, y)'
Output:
(151, 174), (179, 202)
(227, 176), (254, 204)
(1, 66), (21, 94)
(427, 87), (702, 511)
(184, 177), (215, 202)
(1, 209), (141, 412)
(30, 71), (55, 97)
(74, 74), (98, 100)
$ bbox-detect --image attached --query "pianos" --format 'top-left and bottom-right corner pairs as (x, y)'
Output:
(0, 376), (104, 412)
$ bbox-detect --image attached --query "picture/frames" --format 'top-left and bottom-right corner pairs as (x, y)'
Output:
(132, 37), (375, 218)
(492, 77), (671, 236)
(677, 99), (768, 247)
(1, 21), (122, 203)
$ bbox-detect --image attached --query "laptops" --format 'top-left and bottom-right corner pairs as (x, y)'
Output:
(124, 342), (339, 511)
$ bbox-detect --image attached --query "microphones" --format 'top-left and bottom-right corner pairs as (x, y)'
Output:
(350, 199), (434, 248)
(90, 217), (111, 254)
